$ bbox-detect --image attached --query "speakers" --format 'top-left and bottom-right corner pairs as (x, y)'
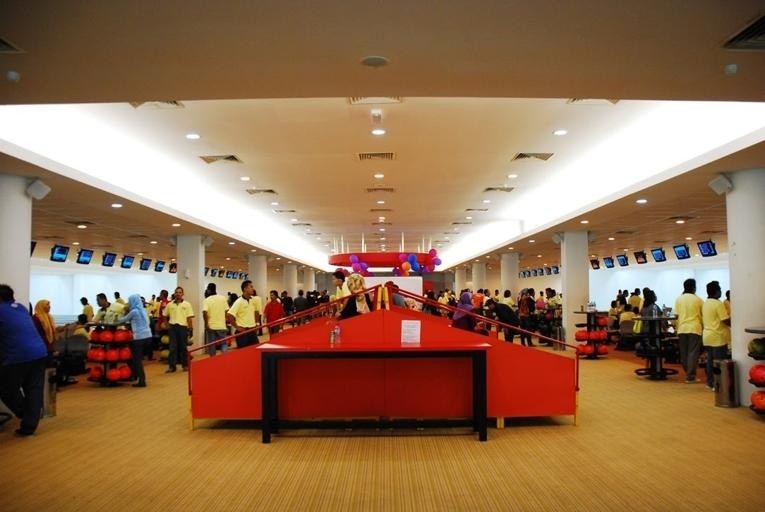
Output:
(708, 175), (732, 196)
(26, 180), (51, 200)
(552, 234), (561, 244)
(588, 231), (597, 241)
(203, 236), (214, 247)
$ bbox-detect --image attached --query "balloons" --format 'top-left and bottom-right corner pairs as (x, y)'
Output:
(392, 248), (442, 276)
(336, 255), (369, 277)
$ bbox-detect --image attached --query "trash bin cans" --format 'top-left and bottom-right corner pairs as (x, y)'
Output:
(551, 326), (566, 351)
(41, 367), (57, 418)
(712, 359), (740, 408)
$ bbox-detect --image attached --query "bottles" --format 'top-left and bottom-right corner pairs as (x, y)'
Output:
(586, 301), (596, 312)
(109, 309), (113, 324)
(662, 303), (666, 316)
(652, 303), (658, 317)
(334, 324), (342, 343)
(101, 309), (106, 323)
(666, 310), (671, 316)
(580, 303), (584, 312)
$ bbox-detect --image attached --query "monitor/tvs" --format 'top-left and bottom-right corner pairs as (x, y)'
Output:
(520, 266), (559, 278)
(205, 267), (249, 280)
(591, 241), (717, 270)
(31, 240), (177, 273)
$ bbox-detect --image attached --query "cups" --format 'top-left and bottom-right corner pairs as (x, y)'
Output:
(330, 331), (335, 343)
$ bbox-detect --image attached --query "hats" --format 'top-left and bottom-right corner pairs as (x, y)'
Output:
(484, 299), (494, 306)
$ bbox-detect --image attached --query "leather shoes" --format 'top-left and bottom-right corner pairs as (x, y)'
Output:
(14, 427), (34, 438)
(166, 367), (176, 373)
(183, 366), (188, 371)
(132, 382), (147, 387)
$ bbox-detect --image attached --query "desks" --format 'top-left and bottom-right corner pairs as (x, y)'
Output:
(632, 315), (678, 381)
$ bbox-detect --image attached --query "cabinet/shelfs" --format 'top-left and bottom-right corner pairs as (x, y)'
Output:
(745, 327), (765, 419)
(87, 322), (136, 387)
(574, 311), (609, 359)
(538, 307), (562, 342)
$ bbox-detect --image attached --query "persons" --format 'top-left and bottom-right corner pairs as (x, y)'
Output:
(1, 281), (78, 436)
(202, 280), (331, 357)
(422, 288), (563, 347)
(608, 279), (731, 392)
(330, 271), (414, 321)
(72, 286), (195, 386)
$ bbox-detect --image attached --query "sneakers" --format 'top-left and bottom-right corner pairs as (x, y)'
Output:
(684, 375), (702, 383)
(705, 385), (713, 390)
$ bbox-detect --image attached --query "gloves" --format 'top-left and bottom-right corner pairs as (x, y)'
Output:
(189, 328), (193, 338)
